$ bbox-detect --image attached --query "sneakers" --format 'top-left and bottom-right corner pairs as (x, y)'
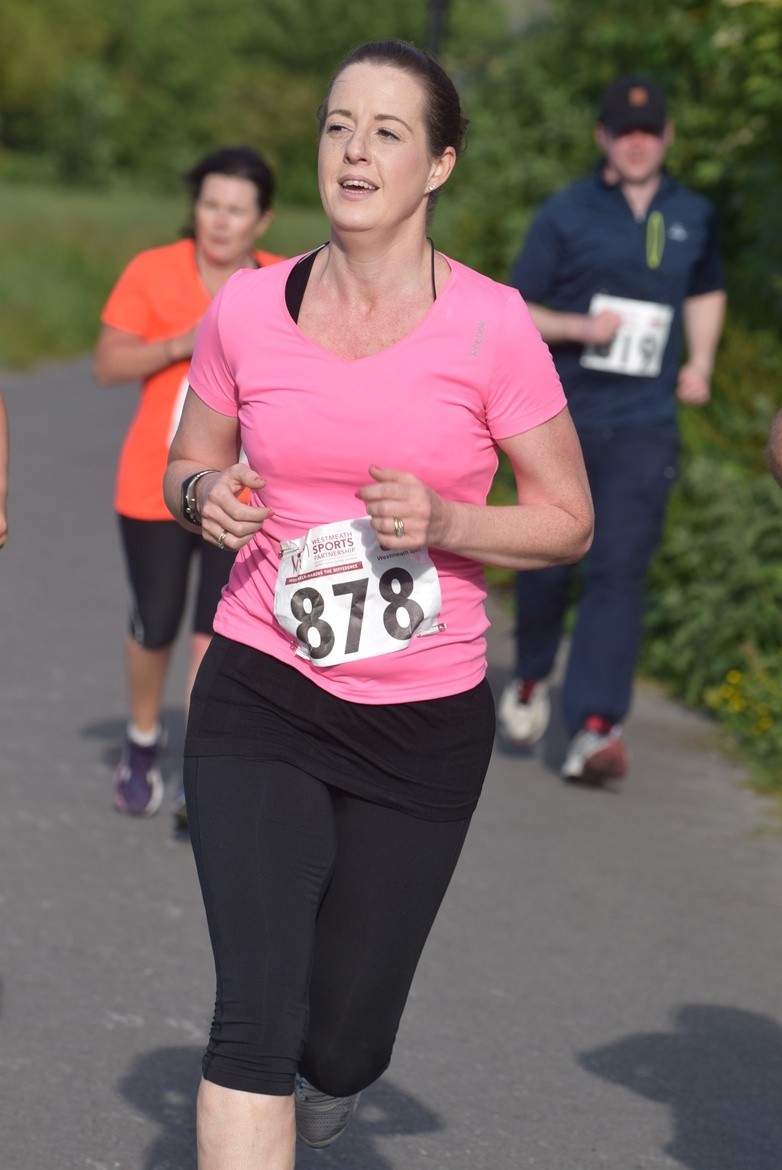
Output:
(496, 677), (551, 748)
(559, 716), (626, 788)
(295, 1072), (362, 1149)
(173, 784), (190, 836)
(113, 717), (165, 818)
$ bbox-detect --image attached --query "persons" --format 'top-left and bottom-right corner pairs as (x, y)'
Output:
(90, 145), (290, 825)
(163, 42), (595, 1170)
(0, 392), (10, 546)
(497, 80), (726, 788)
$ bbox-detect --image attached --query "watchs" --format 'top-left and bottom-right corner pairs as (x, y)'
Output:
(180, 470), (221, 526)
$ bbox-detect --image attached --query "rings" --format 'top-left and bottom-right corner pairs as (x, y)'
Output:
(216, 529), (228, 549)
(393, 516), (404, 537)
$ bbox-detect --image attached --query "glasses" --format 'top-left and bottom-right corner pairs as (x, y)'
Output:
(602, 125), (663, 137)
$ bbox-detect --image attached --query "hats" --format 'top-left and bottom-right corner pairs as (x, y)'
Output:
(597, 80), (666, 136)
